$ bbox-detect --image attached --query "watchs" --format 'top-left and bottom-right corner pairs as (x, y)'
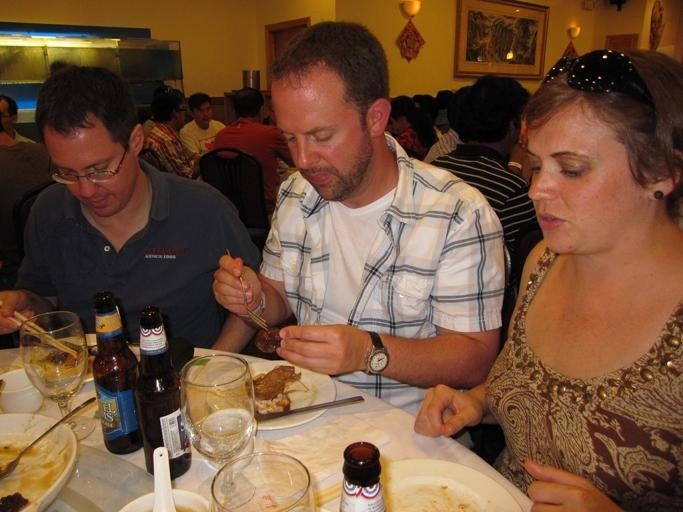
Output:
(364, 328), (389, 380)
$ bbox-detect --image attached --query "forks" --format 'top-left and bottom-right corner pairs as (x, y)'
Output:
(224, 248), (269, 331)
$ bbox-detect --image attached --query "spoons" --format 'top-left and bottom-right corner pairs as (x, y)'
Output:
(154, 447), (177, 511)
(0, 397), (94, 481)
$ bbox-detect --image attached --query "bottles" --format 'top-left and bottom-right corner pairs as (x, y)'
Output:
(92, 290), (144, 456)
(130, 305), (193, 480)
(339, 440), (386, 512)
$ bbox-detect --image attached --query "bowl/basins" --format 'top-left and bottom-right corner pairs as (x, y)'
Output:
(0, 367), (41, 416)
(121, 490), (208, 511)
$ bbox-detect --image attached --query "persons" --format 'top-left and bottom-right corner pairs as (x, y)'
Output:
(389, 94), (438, 161)
(429, 74), (539, 255)
(180, 92), (225, 157)
(212, 21), (505, 454)
(0, 66), (262, 354)
(0, 95), (36, 143)
(141, 89), (200, 180)
(413, 48), (683, 512)
(424, 85), (474, 164)
(0, 120), (57, 291)
(214, 88), (296, 228)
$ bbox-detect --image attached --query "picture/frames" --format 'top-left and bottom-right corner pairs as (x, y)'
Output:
(453, 1), (550, 82)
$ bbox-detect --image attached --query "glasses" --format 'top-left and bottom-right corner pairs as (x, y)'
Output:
(176, 107), (189, 115)
(537, 48), (658, 144)
(45, 145), (130, 184)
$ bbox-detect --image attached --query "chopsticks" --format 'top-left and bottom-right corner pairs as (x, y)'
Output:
(1, 302), (76, 363)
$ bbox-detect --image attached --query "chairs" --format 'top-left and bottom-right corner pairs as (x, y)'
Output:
(13, 179), (59, 277)
(199, 146), (272, 245)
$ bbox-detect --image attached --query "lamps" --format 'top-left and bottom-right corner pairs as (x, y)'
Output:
(568, 24), (581, 41)
(402, 0), (421, 19)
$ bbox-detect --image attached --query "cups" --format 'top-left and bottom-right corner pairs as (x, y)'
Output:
(211, 453), (315, 512)
(180, 353), (254, 508)
(21, 311), (96, 442)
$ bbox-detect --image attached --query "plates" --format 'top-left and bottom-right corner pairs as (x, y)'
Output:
(0, 414), (77, 512)
(380, 459), (521, 511)
(208, 360), (335, 429)
